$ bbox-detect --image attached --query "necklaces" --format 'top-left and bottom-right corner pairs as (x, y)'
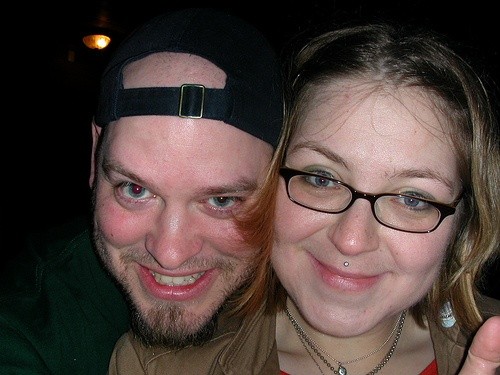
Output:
(284, 307), (407, 375)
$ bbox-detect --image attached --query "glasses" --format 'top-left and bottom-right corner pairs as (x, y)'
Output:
(278, 167), (466, 234)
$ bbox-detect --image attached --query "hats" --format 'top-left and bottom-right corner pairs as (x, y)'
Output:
(95, 8), (285, 146)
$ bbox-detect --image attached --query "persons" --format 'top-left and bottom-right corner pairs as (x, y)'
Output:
(107, 24), (500, 375)
(0, 10), (284, 375)
